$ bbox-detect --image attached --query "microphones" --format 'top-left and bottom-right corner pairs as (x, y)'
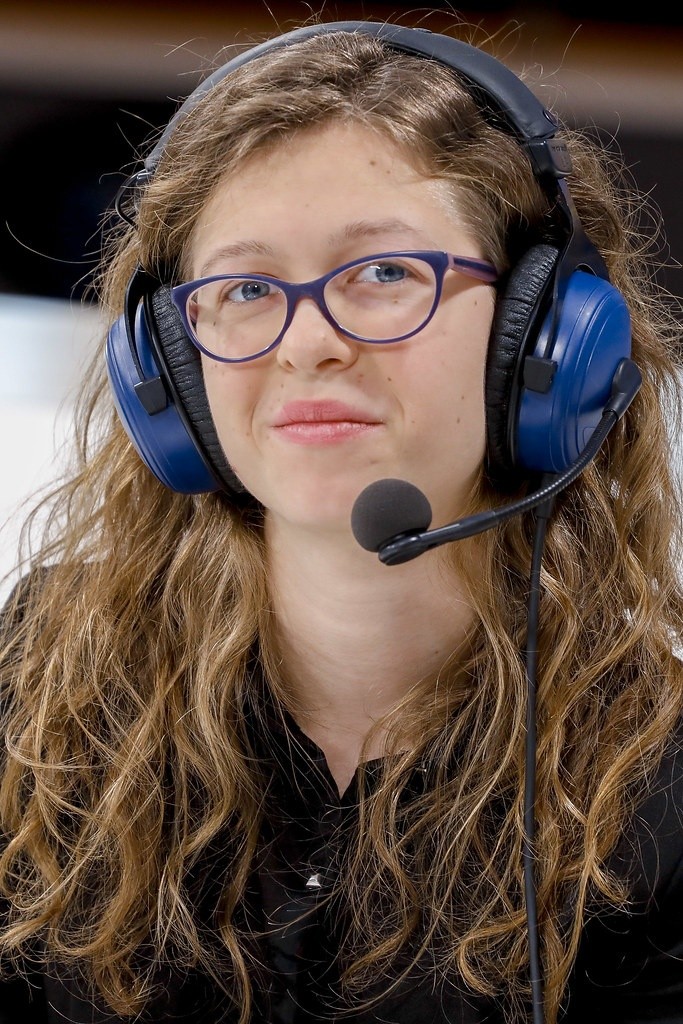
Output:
(351, 356), (640, 565)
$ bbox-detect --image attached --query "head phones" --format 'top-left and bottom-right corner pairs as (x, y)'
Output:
(105, 23), (634, 510)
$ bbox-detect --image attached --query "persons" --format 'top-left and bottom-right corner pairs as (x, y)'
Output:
(0, 17), (683, 1024)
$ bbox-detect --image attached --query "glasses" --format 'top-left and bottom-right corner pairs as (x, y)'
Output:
(169, 246), (504, 365)
